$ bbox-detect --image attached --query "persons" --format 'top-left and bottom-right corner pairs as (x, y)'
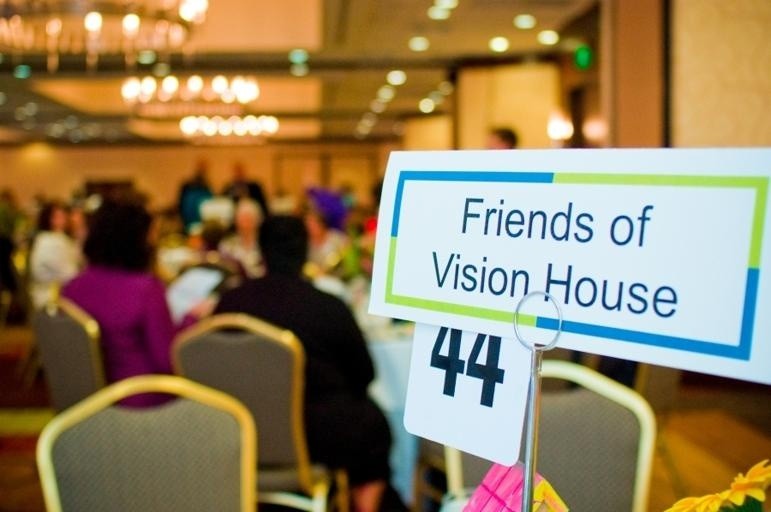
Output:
(489, 130), (516, 148)
(1, 161), (396, 512)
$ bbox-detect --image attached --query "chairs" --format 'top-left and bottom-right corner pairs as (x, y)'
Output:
(33, 376), (259, 512)
(446, 356), (657, 512)
(30, 299), (106, 417)
(10, 252), (373, 325)
(171, 313), (328, 511)
